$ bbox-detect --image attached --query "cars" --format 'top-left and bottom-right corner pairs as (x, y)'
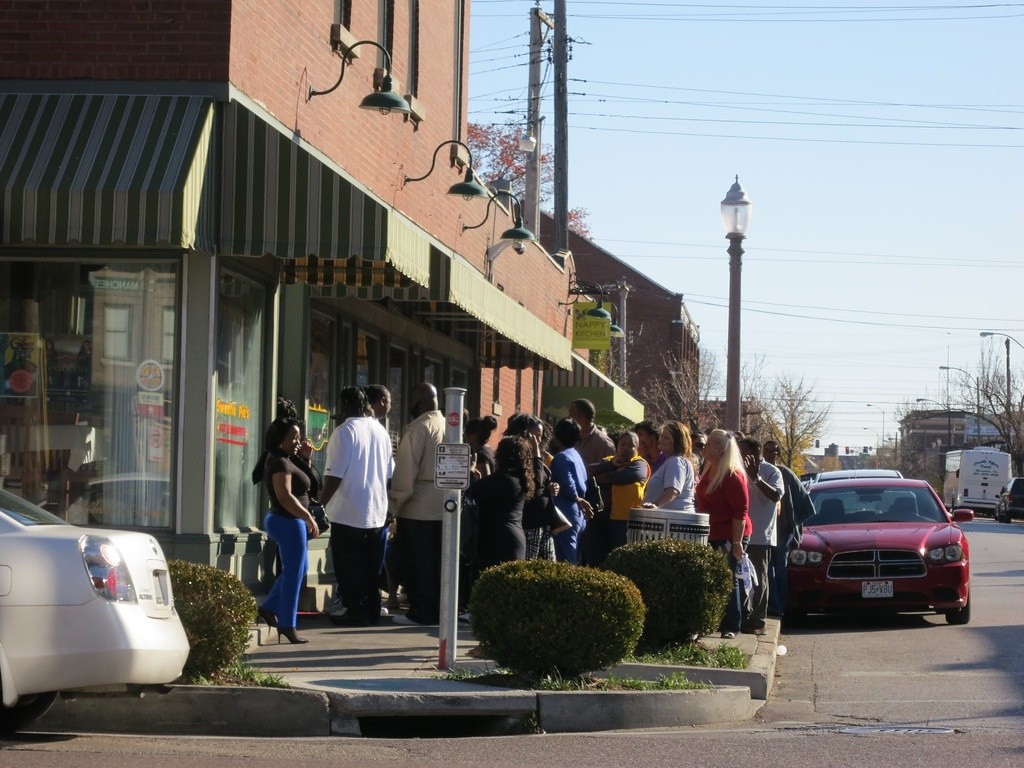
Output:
(39, 474), (171, 528)
(0, 488), (190, 738)
(784, 478), (974, 626)
(814, 468), (905, 484)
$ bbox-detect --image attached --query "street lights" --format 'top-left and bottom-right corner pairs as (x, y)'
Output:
(720, 171), (751, 432)
(939, 365), (982, 446)
(864, 427), (879, 469)
(867, 403), (884, 469)
(916, 398), (952, 451)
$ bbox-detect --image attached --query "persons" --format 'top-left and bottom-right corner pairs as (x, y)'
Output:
(458, 398), (617, 626)
(387, 383), (447, 626)
(588, 419), (816, 639)
(252, 419), (319, 642)
(317, 386), (408, 626)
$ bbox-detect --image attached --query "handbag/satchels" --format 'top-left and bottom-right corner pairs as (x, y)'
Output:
(307, 498), (329, 541)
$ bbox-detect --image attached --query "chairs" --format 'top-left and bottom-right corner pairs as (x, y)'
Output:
(893, 496), (917, 521)
(819, 499), (846, 524)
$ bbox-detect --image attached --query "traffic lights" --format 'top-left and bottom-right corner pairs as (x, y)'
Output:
(863, 447), (866, 452)
(846, 447), (849, 454)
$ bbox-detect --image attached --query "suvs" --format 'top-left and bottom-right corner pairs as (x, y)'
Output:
(994, 477), (1024, 523)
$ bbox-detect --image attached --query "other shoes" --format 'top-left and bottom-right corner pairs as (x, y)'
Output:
(721, 630), (738, 639)
(325, 591), (471, 626)
(741, 621), (766, 635)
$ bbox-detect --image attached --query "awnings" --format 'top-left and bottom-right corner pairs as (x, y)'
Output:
(227, 100), (431, 302)
(0, 93), (218, 256)
(383, 244), (573, 373)
(541, 351), (644, 427)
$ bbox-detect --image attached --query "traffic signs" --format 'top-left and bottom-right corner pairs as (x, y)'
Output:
(435, 442), (470, 490)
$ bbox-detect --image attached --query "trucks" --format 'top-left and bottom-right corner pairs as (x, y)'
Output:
(942, 445), (1012, 518)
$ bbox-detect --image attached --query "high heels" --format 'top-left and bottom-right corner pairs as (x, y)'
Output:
(276, 627), (309, 644)
(256, 606), (278, 628)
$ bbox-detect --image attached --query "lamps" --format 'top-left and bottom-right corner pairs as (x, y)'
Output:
(309, 40), (412, 115)
(462, 192), (535, 245)
(558, 281), (610, 320)
(404, 140), (489, 200)
(610, 303), (625, 337)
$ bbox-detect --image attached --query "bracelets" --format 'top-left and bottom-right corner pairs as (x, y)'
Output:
(753, 476), (762, 484)
